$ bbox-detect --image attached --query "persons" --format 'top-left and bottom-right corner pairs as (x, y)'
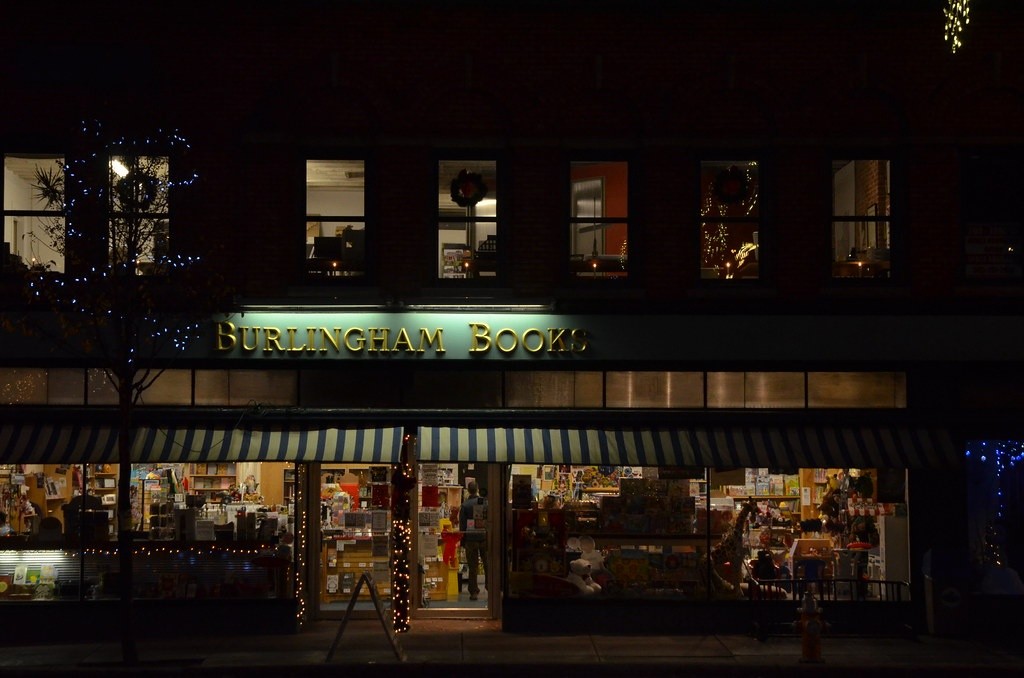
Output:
(461, 482), (490, 600)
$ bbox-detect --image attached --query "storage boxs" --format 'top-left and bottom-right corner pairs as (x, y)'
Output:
(601, 478), (732, 536)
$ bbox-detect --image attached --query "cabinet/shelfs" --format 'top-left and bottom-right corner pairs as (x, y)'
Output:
(11, 462), (238, 540)
(283, 468), (463, 511)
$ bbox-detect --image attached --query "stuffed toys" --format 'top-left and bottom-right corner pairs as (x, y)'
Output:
(697, 495), (759, 598)
(567, 559), (602, 595)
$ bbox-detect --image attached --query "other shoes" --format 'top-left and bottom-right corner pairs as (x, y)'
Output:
(469, 594), (478, 601)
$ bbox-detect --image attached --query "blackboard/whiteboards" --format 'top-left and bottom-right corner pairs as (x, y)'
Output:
(325, 570), (408, 664)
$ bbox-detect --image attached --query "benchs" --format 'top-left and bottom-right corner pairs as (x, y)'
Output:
(746, 578), (917, 643)
